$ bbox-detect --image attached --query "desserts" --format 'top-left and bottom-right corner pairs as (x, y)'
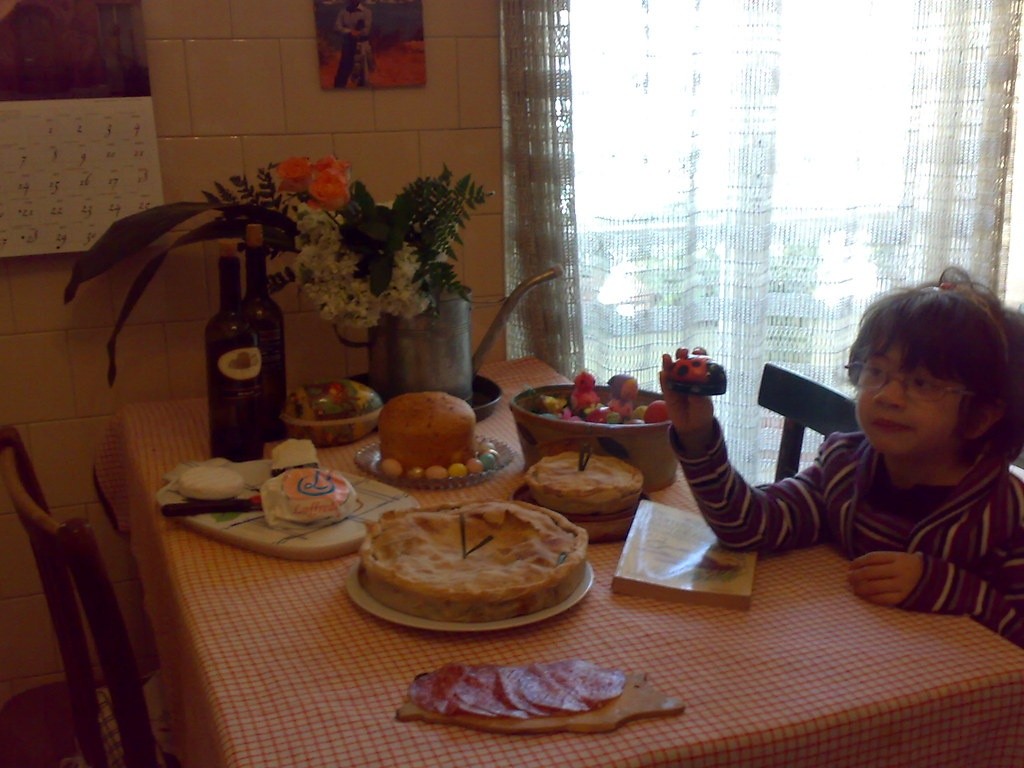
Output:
(260, 439), (358, 526)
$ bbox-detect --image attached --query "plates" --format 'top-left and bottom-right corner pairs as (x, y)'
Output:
(346, 562), (594, 631)
(355, 436), (515, 489)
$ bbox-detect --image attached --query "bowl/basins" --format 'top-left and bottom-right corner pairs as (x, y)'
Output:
(280, 403), (382, 447)
(509, 384), (678, 492)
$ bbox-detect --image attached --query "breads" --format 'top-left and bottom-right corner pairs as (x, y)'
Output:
(526, 452), (643, 514)
(357, 500), (588, 620)
(180, 466), (243, 500)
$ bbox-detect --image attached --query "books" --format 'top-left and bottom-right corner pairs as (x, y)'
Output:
(611, 499), (755, 610)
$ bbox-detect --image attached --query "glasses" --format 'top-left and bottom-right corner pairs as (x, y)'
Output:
(844, 361), (966, 402)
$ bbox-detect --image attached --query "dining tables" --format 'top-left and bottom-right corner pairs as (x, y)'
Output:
(94, 357), (1024, 768)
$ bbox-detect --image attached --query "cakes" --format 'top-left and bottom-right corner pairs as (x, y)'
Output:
(377, 392), (476, 469)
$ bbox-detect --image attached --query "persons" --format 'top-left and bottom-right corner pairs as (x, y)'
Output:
(335, 1), (373, 90)
(658, 266), (1024, 650)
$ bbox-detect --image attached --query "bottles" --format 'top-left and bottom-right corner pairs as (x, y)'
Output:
(241, 248), (285, 442)
(205, 258), (264, 461)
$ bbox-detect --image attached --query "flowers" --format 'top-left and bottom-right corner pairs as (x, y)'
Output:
(64, 156), (495, 390)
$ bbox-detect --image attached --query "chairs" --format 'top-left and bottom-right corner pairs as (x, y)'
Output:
(0, 424), (158, 768)
(757, 363), (857, 482)
